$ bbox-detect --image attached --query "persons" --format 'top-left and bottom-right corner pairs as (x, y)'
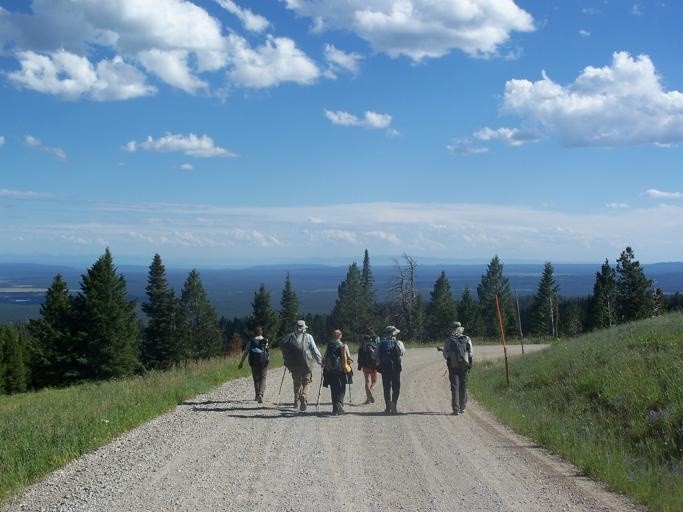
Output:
(443, 322), (472, 415)
(280, 320), (322, 411)
(370, 326), (405, 415)
(321, 329), (353, 415)
(358, 335), (378, 403)
(238, 327), (270, 403)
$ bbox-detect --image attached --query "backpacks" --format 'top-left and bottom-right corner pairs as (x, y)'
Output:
(449, 335), (470, 368)
(380, 341), (402, 373)
(364, 343), (378, 366)
(323, 343), (343, 373)
(280, 334), (314, 372)
(249, 337), (270, 366)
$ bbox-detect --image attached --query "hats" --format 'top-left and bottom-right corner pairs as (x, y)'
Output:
(332, 330), (341, 339)
(447, 322), (464, 336)
(385, 326), (400, 337)
(366, 328), (376, 335)
(294, 320), (307, 331)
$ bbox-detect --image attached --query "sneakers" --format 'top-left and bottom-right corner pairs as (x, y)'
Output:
(300, 395), (307, 411)
(365, 394), (375, 403)
(386, 402), (396, 414)
(451, 410), (464, 415)
(256, 394), (263, 402)
(333, 402), (344, 415)
